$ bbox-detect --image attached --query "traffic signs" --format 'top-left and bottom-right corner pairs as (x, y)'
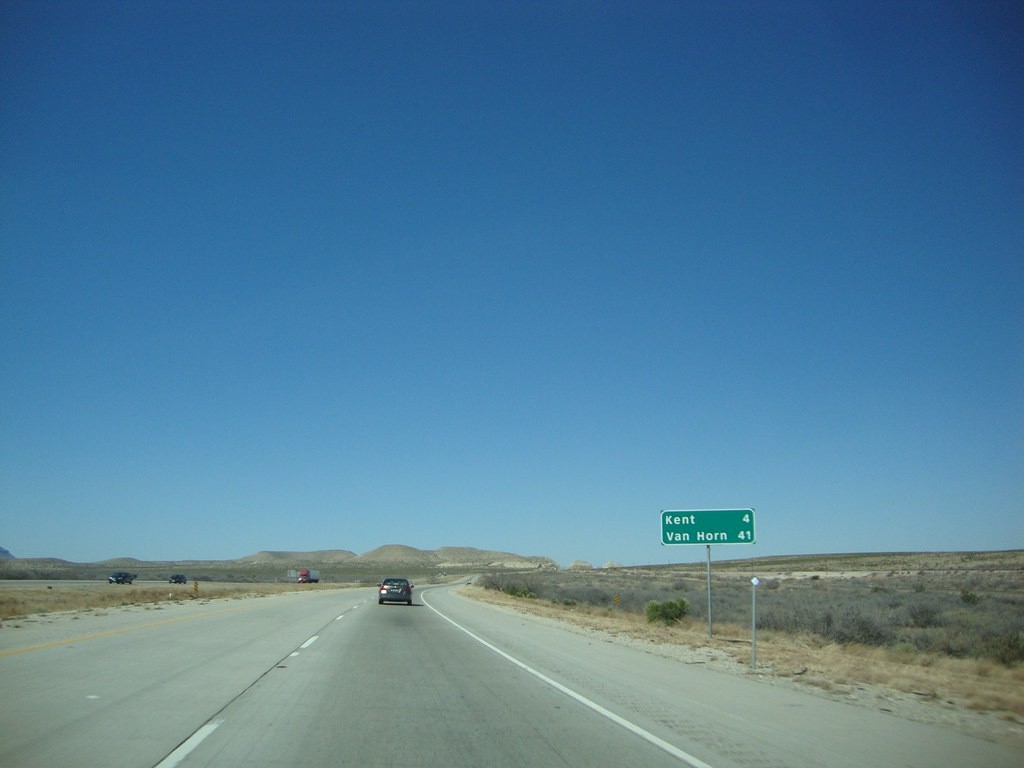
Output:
(660, 507), (756, 546)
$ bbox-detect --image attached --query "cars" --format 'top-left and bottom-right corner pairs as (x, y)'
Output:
(109, 571), (135, 584)
(170, 575), (186, 585)
(378, 576), (414, 605)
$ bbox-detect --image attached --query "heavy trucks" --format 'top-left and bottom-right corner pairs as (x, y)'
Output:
(297, 569), (319, 584)
